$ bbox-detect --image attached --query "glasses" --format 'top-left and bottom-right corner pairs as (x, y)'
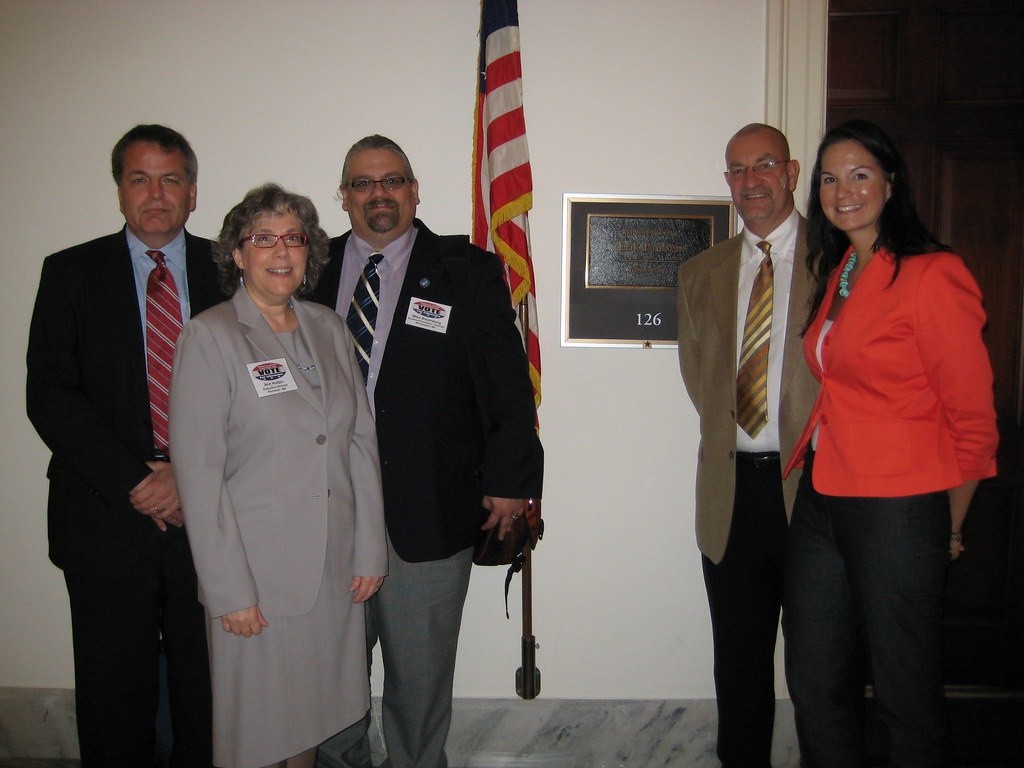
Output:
(344, 176), (411, 193)
(727, 158), (788, 178)
(239, 232), (309, 248)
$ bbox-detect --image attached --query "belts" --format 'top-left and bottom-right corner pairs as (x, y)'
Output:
(737, 452), (782, 480)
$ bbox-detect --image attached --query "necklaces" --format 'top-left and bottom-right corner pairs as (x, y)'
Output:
(282, 298), (315, 371)
(839, 252), (856, 299)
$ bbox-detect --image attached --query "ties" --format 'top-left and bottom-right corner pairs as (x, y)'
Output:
(345, 252), (385, 388)
(143, 250), (184, 453)
(737, 239), (774, 438)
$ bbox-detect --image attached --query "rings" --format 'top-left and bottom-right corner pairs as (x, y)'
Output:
(512, 515), (517, 520)
(154, 505), (161, 512)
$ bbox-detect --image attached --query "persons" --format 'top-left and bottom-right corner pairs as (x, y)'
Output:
(308, 134), (543, 768)
(675, 122), (813, 768)
(782, 125), (998, 768)
(27, 124), (223, 768)
(167, 180), (389, 768)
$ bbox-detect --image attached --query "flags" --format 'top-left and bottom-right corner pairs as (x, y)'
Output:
(471, 0), (545, 432)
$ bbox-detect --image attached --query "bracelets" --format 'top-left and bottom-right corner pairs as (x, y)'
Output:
(951, 532), (962, 541)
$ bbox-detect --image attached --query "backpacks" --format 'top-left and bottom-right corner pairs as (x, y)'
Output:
(472, 417), (544, 619)
